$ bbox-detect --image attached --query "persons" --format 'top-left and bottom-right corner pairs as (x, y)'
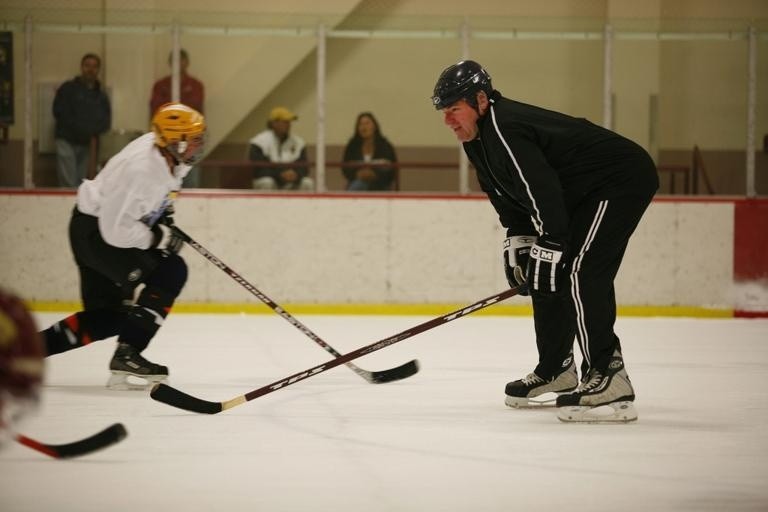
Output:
(245, 107), (314, 192)
(432, 61), (660, 423)
(342, 111), (400, 191)
(36, 102), (207, 390)
(149, 48), (204, 122)
(52, 54), (111, 190)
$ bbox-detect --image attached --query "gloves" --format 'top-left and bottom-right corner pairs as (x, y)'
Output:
(151, 223), (184, 259)
(503, 229), (540, 296)
(527, 236), (573, 297)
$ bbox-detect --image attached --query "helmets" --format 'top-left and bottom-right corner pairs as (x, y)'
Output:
(431, 61), (493, 116)
(151, 102), (207, 166)
(0, 287), (44, 393)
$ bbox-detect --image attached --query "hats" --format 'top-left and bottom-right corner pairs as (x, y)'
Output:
(268, 109), (300, 121)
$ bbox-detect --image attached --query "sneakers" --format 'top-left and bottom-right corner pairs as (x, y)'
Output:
(506, 353), (579, 397)
(110, 345), (168, 376)
(557, 347), (635, 407)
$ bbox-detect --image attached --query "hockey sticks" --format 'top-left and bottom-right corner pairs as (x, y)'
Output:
(147, 280), (529, 415)
(15, 423), (129, 461)
(169, 224), (425, 383)
